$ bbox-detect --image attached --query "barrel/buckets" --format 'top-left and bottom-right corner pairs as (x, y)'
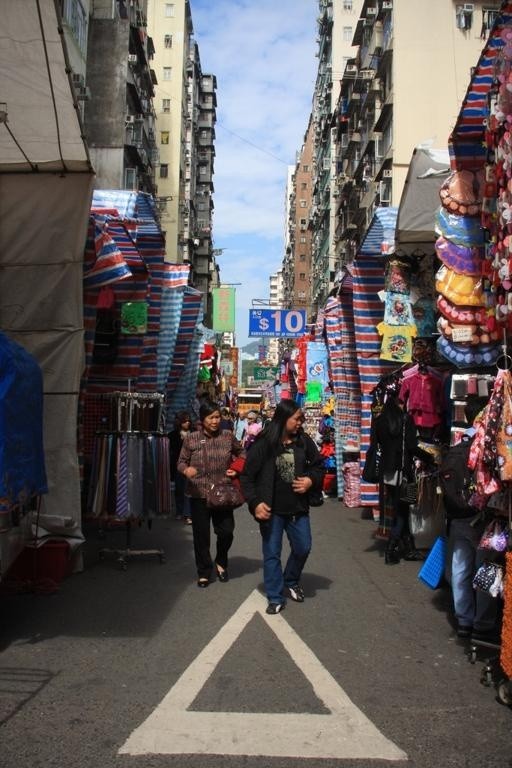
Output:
(25, 541), (69, 592)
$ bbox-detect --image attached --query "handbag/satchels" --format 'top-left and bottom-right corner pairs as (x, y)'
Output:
(362, 442), (385, 483)
(419, 536), (448, 589)
(439, 432), (488, 518)
(473, 564), (503, 598)
(205, 476), (245, 508)
(304, 463), (324, 506)
(480, 520), (508, 553)
(400, 481), (418, 504)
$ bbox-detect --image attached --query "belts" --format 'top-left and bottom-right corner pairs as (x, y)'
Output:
(111, 393), (164, 433)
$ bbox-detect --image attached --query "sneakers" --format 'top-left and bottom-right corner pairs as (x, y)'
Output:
(265, 603), (283, 615)
(289, 584), (305, 602)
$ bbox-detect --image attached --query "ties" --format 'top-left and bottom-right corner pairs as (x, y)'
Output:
(88, 434), (170, 520)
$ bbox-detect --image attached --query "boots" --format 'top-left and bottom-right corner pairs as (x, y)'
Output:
(385, 535), (409, 563)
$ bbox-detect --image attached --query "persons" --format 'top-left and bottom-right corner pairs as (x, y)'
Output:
(169, 401), (282, 524)
(176, 401), (248, 589)
(371, 388), (433, 565)
(238, 397), (326, 617)
(436, 393), (504, 640)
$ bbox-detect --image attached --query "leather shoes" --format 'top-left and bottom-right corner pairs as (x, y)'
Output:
(216, 559), (228, 582)
(197, 578), (208, 588)
